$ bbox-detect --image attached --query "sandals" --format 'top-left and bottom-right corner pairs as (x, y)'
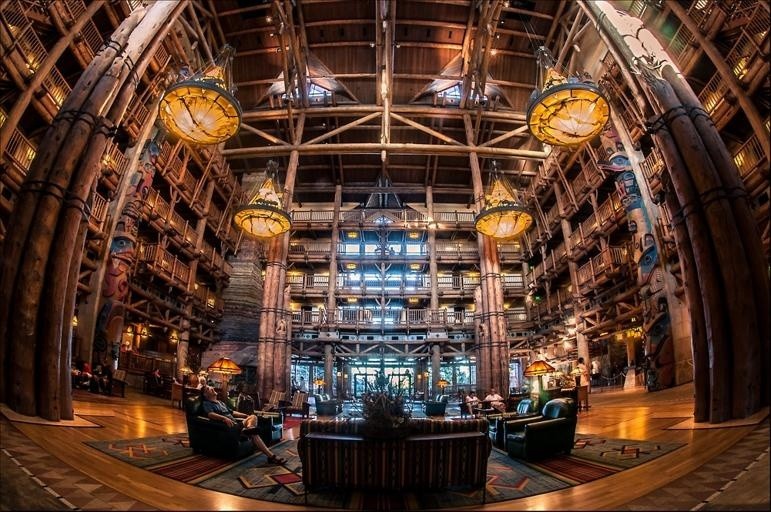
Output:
(241, 426), (259, 436)
(268, 454), (288, 464)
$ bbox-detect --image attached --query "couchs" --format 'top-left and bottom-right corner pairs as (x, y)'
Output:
(323, 393), (345, 413)
(191, 392), (259, 461)
(424, 395), (451, 416)
(299, 417), (494, 512)
(504, 397), (578, 461)
(315, 393), (339, 415)
(141, 371), (175, 395)
(421, 394), (443, 412)
(486, 398), (536, 445)
(237, 399), (285, 440)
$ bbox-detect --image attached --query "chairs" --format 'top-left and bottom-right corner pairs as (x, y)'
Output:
(260, 390), (286, 414)
(459, 393), (482, 419)
(170, 383), (185, 408)
(561, 386), (590, 413)
(284, 392), (312, 418)
(485, 392), (508, 413)
(94, 369), (129, 396)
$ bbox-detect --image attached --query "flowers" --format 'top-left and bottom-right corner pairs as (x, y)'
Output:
(351, 368), (418, 428)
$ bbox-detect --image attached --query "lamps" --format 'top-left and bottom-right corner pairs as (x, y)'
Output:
(518, 10), (611, 147)
(472, 93), (536, 240)
(233, 83), (293, 238)
(208, 355), (242, 400)
(158, 0), (266, 144)
(606, 326), (645, 343)
(177, 365), (193, 379)
(435, 379), (451, 395)
(524, 359), (556, 393)
(570, 367), (587, 386)
(73, 308), (183, 355)
(312, 379), (328, 394)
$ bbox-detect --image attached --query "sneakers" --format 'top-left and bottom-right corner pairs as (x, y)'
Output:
(578, 405), (591, 409)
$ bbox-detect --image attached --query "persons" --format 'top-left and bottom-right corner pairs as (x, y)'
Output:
(153, 367), (161, 381)
(466, 391), (483, 419)
(198, 377), (213, 389)
(73, 359), (114, 395)
(486, 388), (507, 412)
(575, 357), (592, 407)
(201, 384), (289, 465)
(299, 376), (308, 392)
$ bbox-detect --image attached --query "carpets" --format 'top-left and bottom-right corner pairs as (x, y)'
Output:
(84, 420), (690, 511)
